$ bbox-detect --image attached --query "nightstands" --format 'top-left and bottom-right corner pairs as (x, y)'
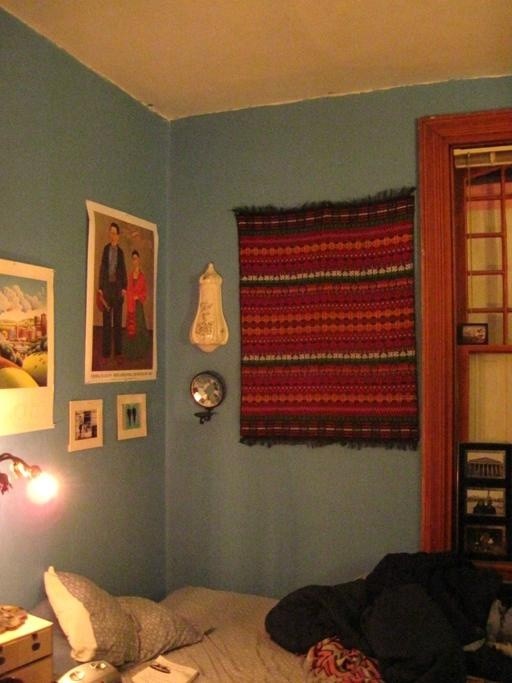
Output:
(1, 610), (54, 683)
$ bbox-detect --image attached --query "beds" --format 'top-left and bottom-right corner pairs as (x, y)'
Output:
(53, 583), (511, 683)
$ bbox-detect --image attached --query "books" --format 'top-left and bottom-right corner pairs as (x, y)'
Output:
(132, 656), (199, 683)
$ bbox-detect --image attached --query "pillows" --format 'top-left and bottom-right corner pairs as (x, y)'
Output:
(44, 564), (204, 660)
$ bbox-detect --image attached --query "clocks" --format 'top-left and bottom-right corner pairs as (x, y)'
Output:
(189, 372), (225, 409)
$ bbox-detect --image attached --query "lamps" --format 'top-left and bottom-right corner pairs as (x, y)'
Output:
(0, 451), (60, 507)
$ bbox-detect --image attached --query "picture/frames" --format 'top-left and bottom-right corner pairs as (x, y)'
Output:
(457, 483), (512, 523)
(456, 323), (487, 345)
(68, 398), (103, 453)
(115, 391), (147, 441)
(458, 443), (512, 482)
(455, 519), (512, 561)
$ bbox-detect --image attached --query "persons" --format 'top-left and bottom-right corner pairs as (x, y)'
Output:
(125, 249), (148, 357)
(473, 500), (485, 514)
(485, 496), (496, 514)
(99, 222), (127, 354)
(80, 411), (92, 438)
(127, 407), (131, 422)
(133, 407), (136, 422)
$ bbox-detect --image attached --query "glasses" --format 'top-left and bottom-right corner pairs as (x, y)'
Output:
(149, 662), (171, 673)
